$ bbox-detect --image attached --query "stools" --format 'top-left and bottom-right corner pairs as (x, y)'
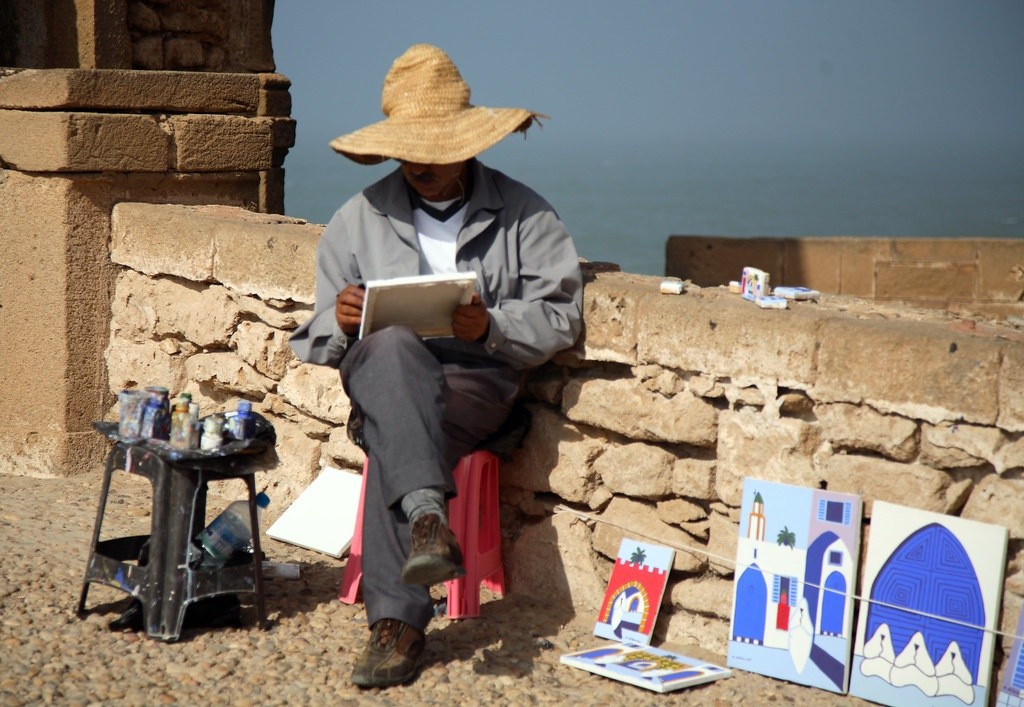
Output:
(337, 447), (507, 619)
(74, 442), (271, 641)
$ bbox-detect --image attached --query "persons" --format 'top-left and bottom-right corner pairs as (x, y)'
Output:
(288, 44), (583, 688)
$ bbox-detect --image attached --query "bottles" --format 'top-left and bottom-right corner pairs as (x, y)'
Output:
(190, 492), (271, 569)
(139, 537), (151, 567)
(168, 393), (199, 450)
(228, 402), (252, 441)
(261, 561), (300, 580)
(200, 414), (223, 452)
(140, 398), (170, 439)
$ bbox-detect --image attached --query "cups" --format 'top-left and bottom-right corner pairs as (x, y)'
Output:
(144, 386), (169, 411)
(118, 390), (146, 438)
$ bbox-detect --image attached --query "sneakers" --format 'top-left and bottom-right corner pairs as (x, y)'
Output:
(401, 513), (467, 585)
(350, 618), (427, 687)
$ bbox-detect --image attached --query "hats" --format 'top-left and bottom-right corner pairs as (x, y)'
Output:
(328, 44), (552, 165)
